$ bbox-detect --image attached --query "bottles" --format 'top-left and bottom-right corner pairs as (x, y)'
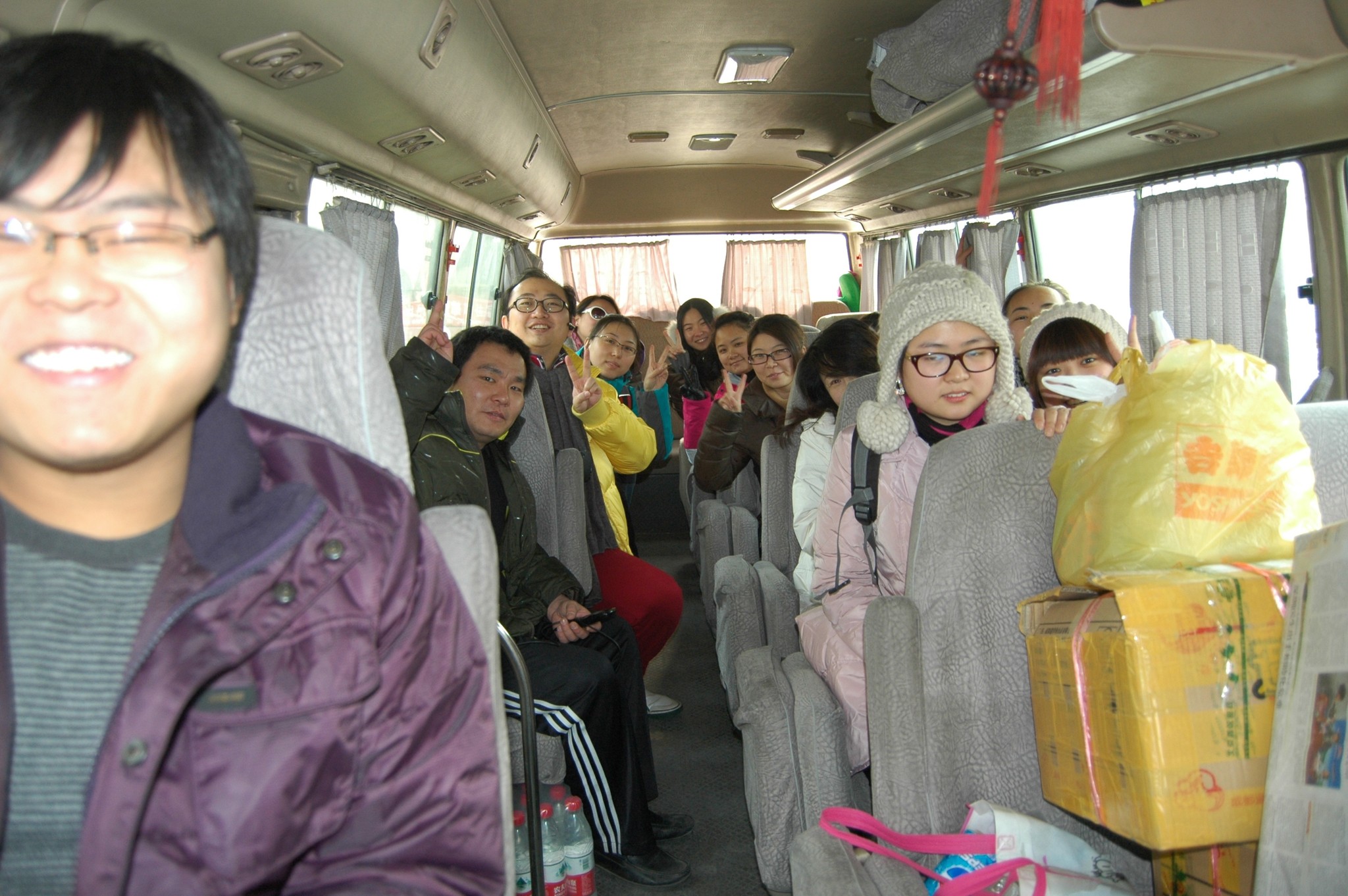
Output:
(511, 782), (594, 896)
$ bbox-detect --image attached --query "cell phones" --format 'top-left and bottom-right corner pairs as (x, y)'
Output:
(568, 607), (616, 628)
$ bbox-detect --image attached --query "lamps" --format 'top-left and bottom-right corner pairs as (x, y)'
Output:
(688, 133), (737, 151)
(713, 44), (793, 83)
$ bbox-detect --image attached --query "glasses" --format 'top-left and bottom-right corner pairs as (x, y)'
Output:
(902, 345), (998, 377)
(592, 335), (636, 356)
(0, 221), (221, 281)
(747, 348), (793, 364)
(581, 307), (617, 322)
(504, 297), (570, 314)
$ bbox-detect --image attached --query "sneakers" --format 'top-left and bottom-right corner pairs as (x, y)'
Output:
(643, 689), (683, 716)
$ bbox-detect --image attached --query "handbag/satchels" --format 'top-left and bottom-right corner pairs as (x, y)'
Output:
(819, 802), (1135, 895)
(1050, 337), (1322, 591)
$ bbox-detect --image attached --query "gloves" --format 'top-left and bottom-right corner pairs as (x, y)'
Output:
(679, 364), (705, 401)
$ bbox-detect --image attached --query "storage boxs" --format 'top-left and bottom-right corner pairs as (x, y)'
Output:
(1151, 838), (1259, 896)
(1013, 553), (1293, 853)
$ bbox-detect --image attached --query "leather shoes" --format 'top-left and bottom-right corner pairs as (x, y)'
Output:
(593, 845), (692, 887)
(649, 813), (696, 844)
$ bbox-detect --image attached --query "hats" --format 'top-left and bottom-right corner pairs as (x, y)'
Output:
(1019, 300), (1130, 382)
(855, 259), (1032, 453)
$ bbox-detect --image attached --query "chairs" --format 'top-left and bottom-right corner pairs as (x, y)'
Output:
(219, 217), (1348, 896)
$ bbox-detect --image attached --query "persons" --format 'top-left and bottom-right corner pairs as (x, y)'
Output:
(575, 314), (674, 506)
(693, 314), (807, 492)
(860, 312), (880, 332)
(1015, 302), (1143, 438)
(792, 318), (880, 614)
(563, 295), (620, 352)
(794, 260), (1071, 776)
(662, 298), (714, 420)
(1002, 279), (1070, 359)
(0, 32), (503, 896)
(501, 267), (686, 717)
(679, 311), (756, 465)
(390, 299), (694, 891)
